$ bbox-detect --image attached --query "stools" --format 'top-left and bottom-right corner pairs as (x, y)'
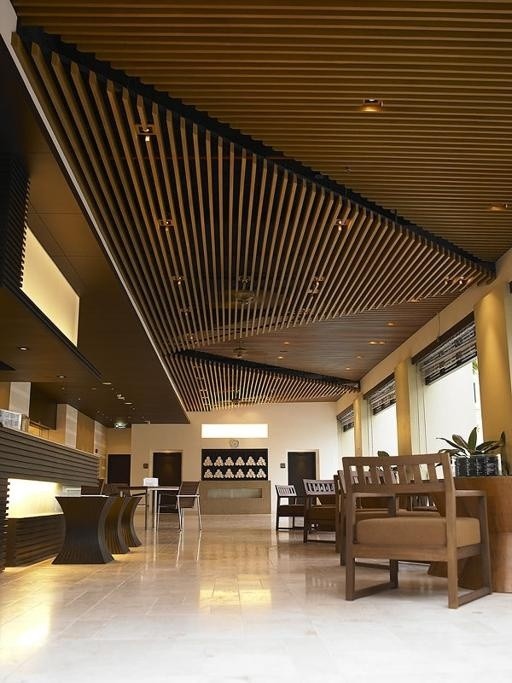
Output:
(52, 494), (143, 564)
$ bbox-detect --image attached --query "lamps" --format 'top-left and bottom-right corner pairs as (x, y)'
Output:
(231, 278), (255, 408)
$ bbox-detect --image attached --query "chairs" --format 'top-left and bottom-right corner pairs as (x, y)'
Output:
(111, 480), (202, 531)
(274, 453), (493, 609)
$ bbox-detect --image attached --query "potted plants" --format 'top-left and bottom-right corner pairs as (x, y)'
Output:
(434, 426), (505, 476)
